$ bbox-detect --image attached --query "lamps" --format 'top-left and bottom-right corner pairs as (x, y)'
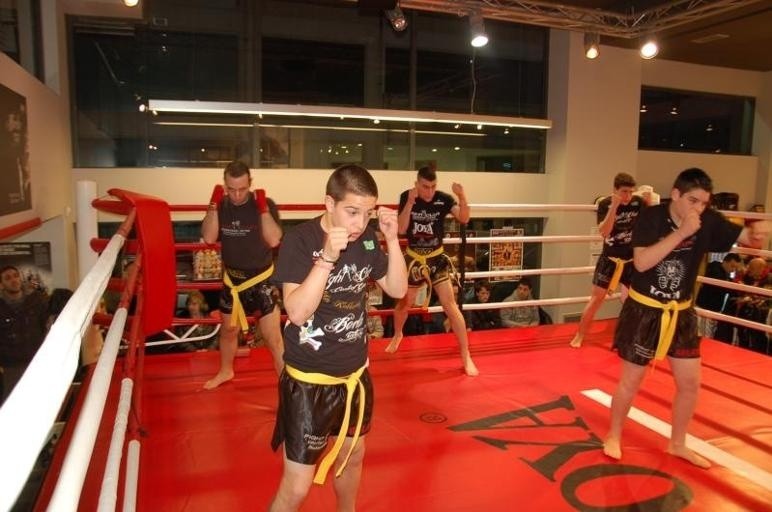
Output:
(467, 5), (490, 49)
(145, 46), (553, 130)
(638, 32), (660, 59)
(385, 5), (407, 32)
(584, 30), (600, 60)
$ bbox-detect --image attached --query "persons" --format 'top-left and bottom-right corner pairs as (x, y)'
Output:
(271, 164), (409, 512)
(0, 267), (541, 404)
(696, 254), (772, 356)
(384, 166), (480, 377)
(569, 173), (652, 348)
(603, 168), (772, 469)
(201, 160), (286, 391)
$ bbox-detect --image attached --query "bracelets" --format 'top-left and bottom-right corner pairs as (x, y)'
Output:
(312, 247), (336, 264)
(312, 257), (335, 270)
